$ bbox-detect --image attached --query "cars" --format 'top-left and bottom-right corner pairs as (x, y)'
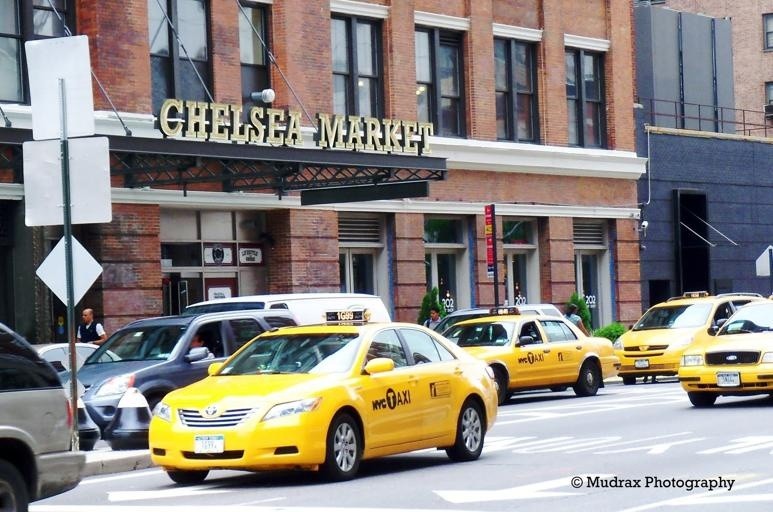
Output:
(148, 322), (498, 484)
(79, 307), (299, 442)
(28, 339), (123, 448)
(435, 302), (620, 406)
(1, 321), (87, 511)
(678, 302), (773, 406)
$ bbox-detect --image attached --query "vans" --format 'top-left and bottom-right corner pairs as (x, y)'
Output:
(613, 289), (764, 385)
(182, 292), (390, 324)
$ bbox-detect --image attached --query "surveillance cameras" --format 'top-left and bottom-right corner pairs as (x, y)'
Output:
(638, 221), (648, 231)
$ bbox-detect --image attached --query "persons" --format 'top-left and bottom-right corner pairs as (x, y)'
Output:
(561, 303), (588, 337)
(189, 332), (213, 358)
(76, 307), (108, 346)
(422, 306), (449, 332)
